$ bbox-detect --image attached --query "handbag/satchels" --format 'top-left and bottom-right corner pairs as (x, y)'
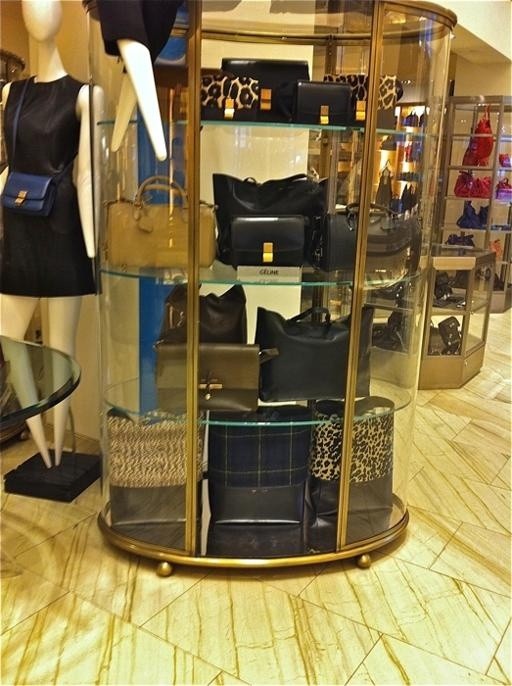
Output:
(307, 396), (395, 517)
(386, 298), (431, 343)
(1, 172), (58, 218)
(174, 58), (396, 128)
(404, 114), (428, 127)
(446, 234), (476, 255)
(462, 118), (494, 166)
(255, 305), (375, 403)
(156, 342), (260, 414)
(434, 273), (452, 300)
(104, 409), (207, 527)
(489, 241), (503, 262)
(439, 317), (461, 348)
(103, 202), (217, 268)
(454, 175), (512, 200)
(208, 405), (312, 524)
(381, 139), (396, 150)
(458, 201), (488, 228)
(163, 284), (247, 343)
(213, 173), (408, 271)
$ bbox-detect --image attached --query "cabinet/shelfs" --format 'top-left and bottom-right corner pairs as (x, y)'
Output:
(359, 241), (495, 389)
(308, 97), (511, 315)
(82, 0), (461, 580)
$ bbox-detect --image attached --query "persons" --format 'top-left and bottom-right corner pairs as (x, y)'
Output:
(97, 0), (182, 161)
(0, 0), (104, 476)
